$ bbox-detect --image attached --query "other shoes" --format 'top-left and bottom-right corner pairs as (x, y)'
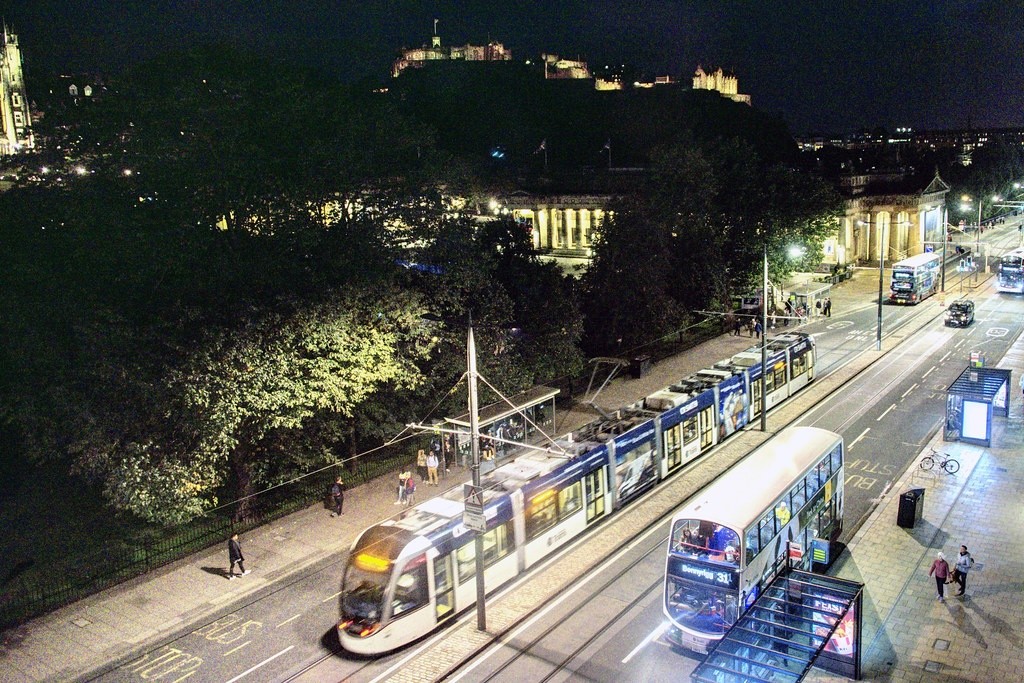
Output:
(959, 587), (962, 591)
(339, 512), (344, 517)
(960, 591), (966, 596)
(230, 576), (236, 580)
(403, 500), (406, 505)
(331, 513), (335, 517)
(936, 594), (940, 599)
(242, 570), (251, 576)
(939, 597), (943, 603)
(394, 501), (401, 504)
(446, 468), (450, 471)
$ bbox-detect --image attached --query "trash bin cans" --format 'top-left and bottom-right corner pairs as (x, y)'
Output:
(897, 488), (927, 528)
(632, 354), (651, 378)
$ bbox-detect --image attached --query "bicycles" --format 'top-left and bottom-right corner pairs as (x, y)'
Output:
(920, 449), (960, 474)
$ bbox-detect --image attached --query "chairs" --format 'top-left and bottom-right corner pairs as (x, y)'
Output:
(723, 594), (737, 617)
(726, 471), (828, 556)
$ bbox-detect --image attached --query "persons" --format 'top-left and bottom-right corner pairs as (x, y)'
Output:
(777, 502), (790, 525)
(784, 298), (792, 325)
(479, 409), (532, 460)
(796, 302), (806, 323)
(947, 234), (952, 249)
(331, 476), (345, 517)
(981, 217), (1005, 233)
(953, 545), (971, 596)
(725, 540), (751, 565)
(537, 410), (546, 425)
(770, 311), (777, 328)
(774, 606), (792, 651)
(834, 263), (839, 273)
(1020, 374), (1024, 394)
(395, 469), (416, 506)
(417, 432), (455, 487)
(816, 297), (832, 318)
(680, 528), (707, 556)
(928, 552), (950, 602)
(228, 533), (251, 580)
(735, 317), (762, 338)
(959, 219), (964, 232)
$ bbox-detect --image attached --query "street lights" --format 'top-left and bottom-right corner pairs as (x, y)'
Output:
(856, 220), (914, 351)
(961, 194), (999, 282)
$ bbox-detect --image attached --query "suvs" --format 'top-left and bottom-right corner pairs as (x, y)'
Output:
(944, 299), (974, 328)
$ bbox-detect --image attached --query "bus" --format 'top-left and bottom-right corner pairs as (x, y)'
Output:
(336, 330), (816, 656)
(889, 252), (941, 305)
(996, 246), (1024, 294)
(662, 426), (846, 657)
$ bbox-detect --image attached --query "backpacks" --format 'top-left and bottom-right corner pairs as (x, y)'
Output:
(332, 485), (340, 497)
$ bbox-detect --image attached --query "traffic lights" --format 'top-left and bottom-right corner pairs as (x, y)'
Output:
(967, 256), (972, 266)
(960, 260), (965, 272)
